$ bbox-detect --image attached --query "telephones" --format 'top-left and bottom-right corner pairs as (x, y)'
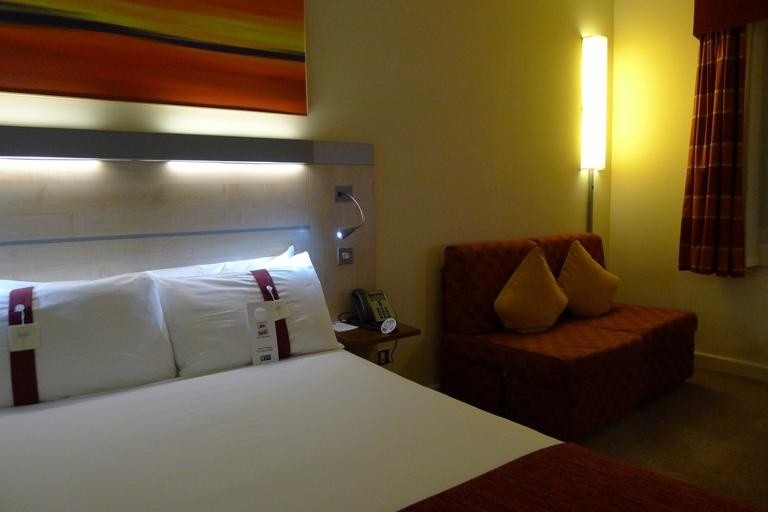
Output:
(351, 288), (397, 323)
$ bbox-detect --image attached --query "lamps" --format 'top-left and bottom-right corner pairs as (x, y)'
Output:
(335, 192), (366, 240)
(579, 30), (608, 233)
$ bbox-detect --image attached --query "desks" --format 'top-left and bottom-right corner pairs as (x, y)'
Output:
(336, 319), (421, 361)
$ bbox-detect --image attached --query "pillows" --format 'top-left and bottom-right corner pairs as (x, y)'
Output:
(488, 239), (622, 334)
(161, 250), (343, 380)
(144, 245), (296, 277)
(3, 271), (175, 405)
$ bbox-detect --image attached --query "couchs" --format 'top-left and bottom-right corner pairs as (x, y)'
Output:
(436, 232), (699, 443)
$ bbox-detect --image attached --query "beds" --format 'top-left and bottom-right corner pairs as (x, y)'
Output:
(2, 345), (766, 510)
